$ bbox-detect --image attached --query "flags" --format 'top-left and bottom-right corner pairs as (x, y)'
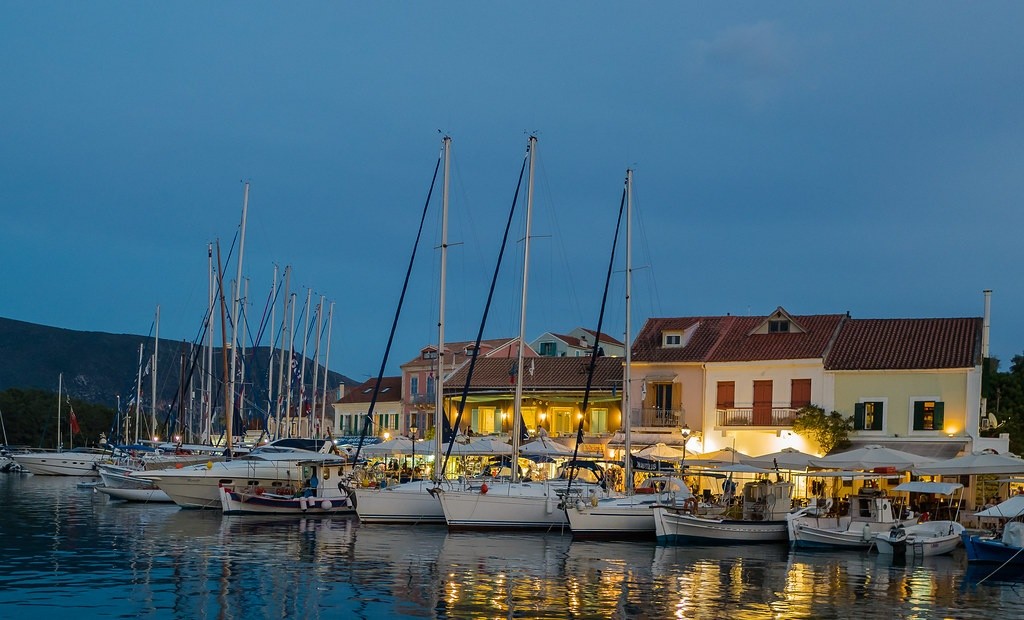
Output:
(70, 407), (80, 439)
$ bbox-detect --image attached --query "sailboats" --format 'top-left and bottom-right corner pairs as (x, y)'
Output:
(0, 127), (1024, 566)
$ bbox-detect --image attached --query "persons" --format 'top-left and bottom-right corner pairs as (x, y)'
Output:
(537, 424), (547, 436)
(919, 495), (929, 521)
(464, 424), (474, 436)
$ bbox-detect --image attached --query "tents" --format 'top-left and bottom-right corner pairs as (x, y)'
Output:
(740, 446), (823, 505)
(630, 442), (690, 496)
(442, 442), (465, 487)
(518, 439), (583, 487)
(810, 442), (938, 519)
(401, 439), (446, 486)
(450, 437), (514, 487)
(681, 449), (752, 504)
(914, 449), (1024, 521)
(363, 435), (421, 486)
